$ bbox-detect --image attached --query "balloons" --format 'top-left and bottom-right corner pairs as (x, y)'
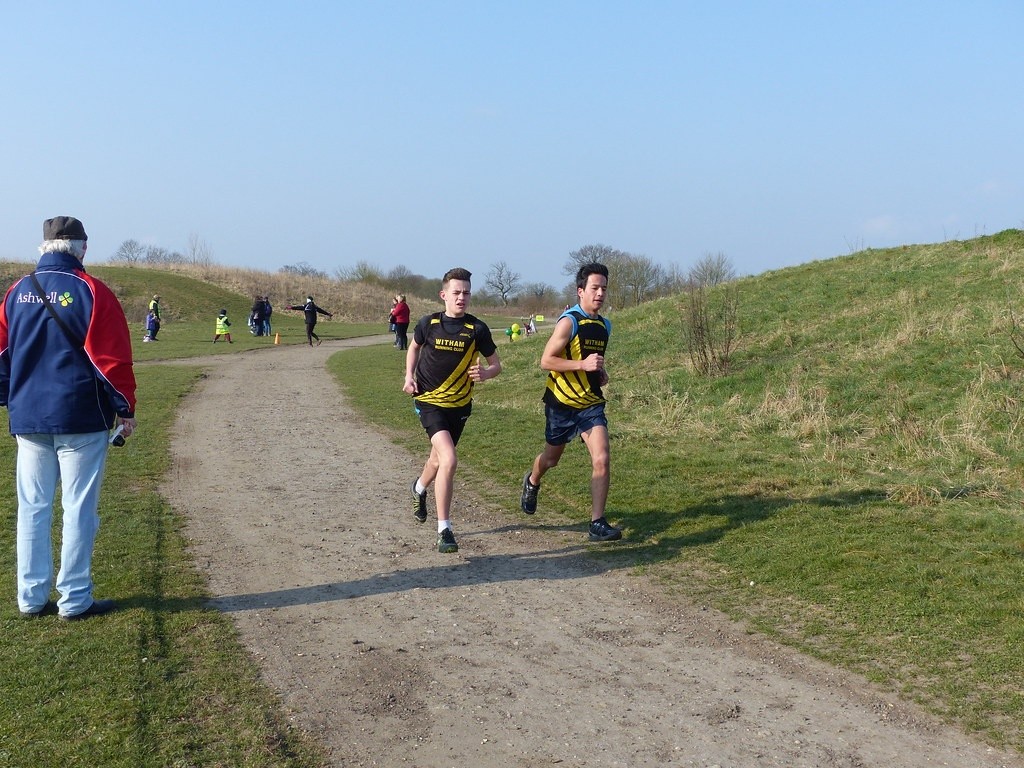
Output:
(506, 323), (521, 342)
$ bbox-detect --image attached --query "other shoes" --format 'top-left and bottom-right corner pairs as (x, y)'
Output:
(318, 340), (322, 346)
(306, 345), (312, 347)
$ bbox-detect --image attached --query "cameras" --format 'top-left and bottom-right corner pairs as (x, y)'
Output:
(108, 424), (125, 447)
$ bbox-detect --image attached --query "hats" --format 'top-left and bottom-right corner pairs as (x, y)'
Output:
(42, 216), (88, 241)
(153, 294), (161, 298)
(307, 296), (313, 301)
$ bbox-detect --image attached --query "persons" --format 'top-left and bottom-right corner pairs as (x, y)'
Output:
(251, 295), (272, 336)
(564, 304), (571, 310)
(149, 294), (162, 337)
(389, 293), (410, 350)
(0, 216), (137, 622)
(402, 268), (502, 554)
(286, 296), (334, 346)
(213, 309), (231, 343)
(147, 309), (158, 341)
(522, 263), (622, 541)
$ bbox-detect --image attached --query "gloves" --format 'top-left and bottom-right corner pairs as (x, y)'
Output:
(287, 306), (291, 309)
(328, 313), (333, 317)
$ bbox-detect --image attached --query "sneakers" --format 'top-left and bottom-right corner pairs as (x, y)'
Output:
(411, 477), (428, 523)
(437, 528), (458, 553)
(590, 517), (622, 541)
(520, 472), (541, 514)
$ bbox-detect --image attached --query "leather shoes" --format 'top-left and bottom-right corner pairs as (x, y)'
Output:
(58, 599), (114, 620)
(21, 600), (58, 618)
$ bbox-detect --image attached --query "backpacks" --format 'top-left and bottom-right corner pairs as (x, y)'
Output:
(265, 302), (272, 315)
